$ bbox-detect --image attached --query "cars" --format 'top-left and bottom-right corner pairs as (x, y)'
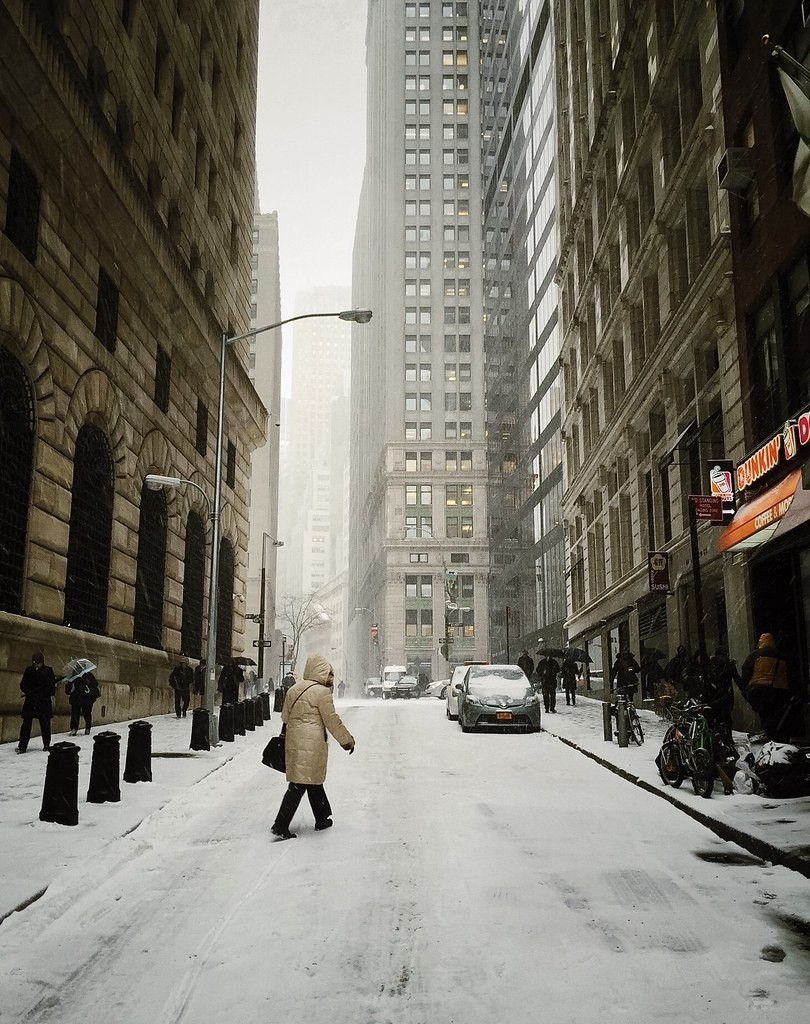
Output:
(445, 664), (478, 721)
(362, 664), (451, 700)
(454, 663), (541, 735)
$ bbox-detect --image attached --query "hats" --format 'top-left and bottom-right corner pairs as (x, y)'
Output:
(32, 652), (44, 662)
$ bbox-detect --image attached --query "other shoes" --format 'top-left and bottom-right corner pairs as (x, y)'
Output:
(85, 727), (92, 735)
(545, 708), (550, 713)
(43, 745), (50, 751)
(551, 707), (558, 714)
(183, 711), (187, 717)
(177, 714), (181, 718)
(315, 819), (333, 830)
(566, 700), (571, 706)
(16, 747), (28, 755)
(71, 729), (78, 736)
(572, 700), (576, 705)
(271, 823), (297, 839)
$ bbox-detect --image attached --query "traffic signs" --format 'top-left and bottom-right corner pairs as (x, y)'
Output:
(687, 494), (723, 522)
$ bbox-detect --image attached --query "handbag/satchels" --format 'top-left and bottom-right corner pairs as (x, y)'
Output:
(261, 737), (286, 773)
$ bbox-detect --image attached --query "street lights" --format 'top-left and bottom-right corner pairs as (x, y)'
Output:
(256, 529), (285, 696)
(403, 524), (471, 663)
(354, 607), (383, 683)
(142, 305), (375, 755)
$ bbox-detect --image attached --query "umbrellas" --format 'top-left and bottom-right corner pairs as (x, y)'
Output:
(536, 648), (563, 670)
(233, 656), (257, 672)
(561, 648), (594, 664)
(58, 657), (97, 693)
(641, 648), (666, 664)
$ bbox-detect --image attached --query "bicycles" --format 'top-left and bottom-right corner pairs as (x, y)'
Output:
(653, 696), (719, 798)
(610, 680), (646, 747)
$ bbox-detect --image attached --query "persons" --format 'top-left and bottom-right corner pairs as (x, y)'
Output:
(338, 681), (345, 697)
(192, 660), (208, 712)
(168, 656), (193, 720)
(270, 655), (355, 840)
(664, 633), (790, 745)
(518, 650), (534, 673)
(216, 658), (244, 705)
(536, 657), (560, 713)
(610, 648), (641, 718)
(281, 672), (296, 695)
(65, 664), (99, 736)
(14, 652), (55, 754)
(268, 678), (274, 692)
(644, 661), (663, 699)
(561, 660), (580, 705)
